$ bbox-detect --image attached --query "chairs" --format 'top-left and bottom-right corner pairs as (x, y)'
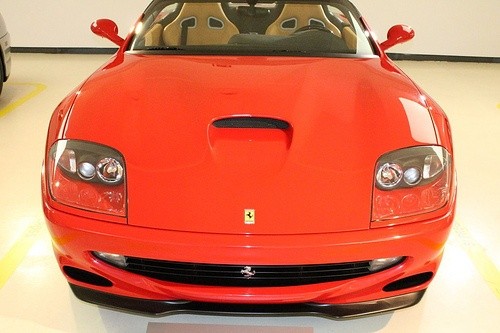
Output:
(264, 0), (342, 40)
(159, 2), (239, 49)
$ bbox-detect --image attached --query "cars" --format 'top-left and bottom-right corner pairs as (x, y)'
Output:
(39, 0), (460, 322)
